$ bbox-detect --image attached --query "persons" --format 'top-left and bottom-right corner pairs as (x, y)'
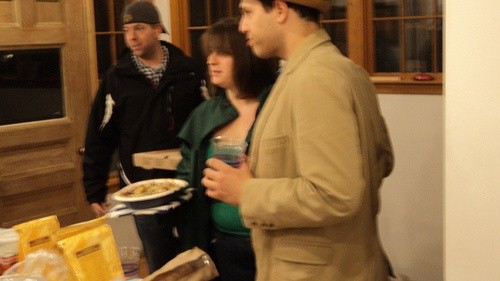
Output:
(202, 0), (394, 281)
(173, 17), (281, 281)
(81, 0), (209, 274)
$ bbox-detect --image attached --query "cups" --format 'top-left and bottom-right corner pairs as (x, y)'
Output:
(212, 137), (249, 170)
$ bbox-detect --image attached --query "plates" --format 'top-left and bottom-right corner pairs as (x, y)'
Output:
(112, 178), (190, 210)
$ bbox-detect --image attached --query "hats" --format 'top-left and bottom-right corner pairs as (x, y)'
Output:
(121, 1), (169, 35)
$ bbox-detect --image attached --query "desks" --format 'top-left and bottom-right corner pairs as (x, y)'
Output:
(133, 149), (182, 179)
